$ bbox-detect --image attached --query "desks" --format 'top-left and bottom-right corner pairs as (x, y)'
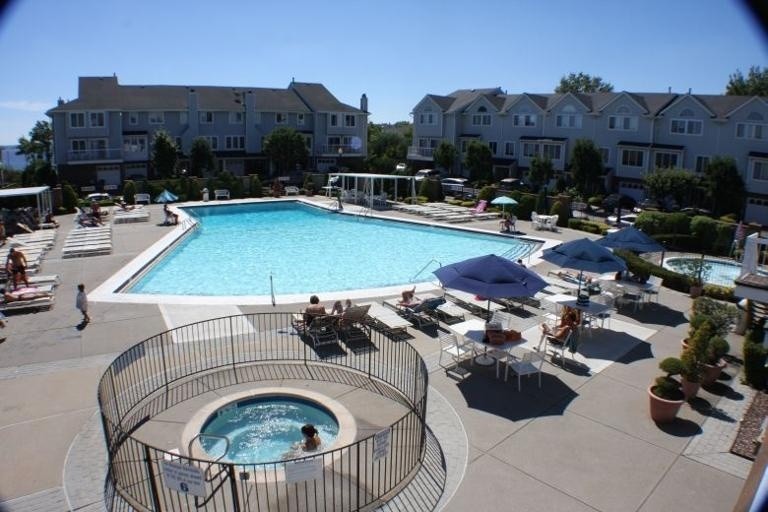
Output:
(465, 329), (527, 378)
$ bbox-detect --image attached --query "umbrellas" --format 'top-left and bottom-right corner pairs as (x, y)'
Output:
(153, 189), (179, 203)
(490, 195), (519, 220)
(539, 224), (668, 296)
(431, 254), (551, 323)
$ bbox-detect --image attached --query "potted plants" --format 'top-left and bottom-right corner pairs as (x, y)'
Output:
(688, 259), (702, 299)
(647, 313), (728, 422)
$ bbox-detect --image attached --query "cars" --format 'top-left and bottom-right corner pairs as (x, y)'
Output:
(396, 163), (407, 170)
(604, 198), (712, 227)
(590, 205), (605, 216)
(79, 192), (111, 201)
(415, 168), (469, 195)
(501, 177), (524, 190)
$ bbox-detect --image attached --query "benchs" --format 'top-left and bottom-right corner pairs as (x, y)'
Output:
(284, 186), (300, 196)
(214, 189), (230, 200)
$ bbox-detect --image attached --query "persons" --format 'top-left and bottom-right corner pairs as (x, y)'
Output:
(0, 198), (137, 327)
(517, 259), (528, 269)
(296, 284), (428, 333)
(163, 205), (178, 224)
(200, 186), (209, 202)
(505, 213), (517, 232)
(290, 424), (321, 452)
(542, 304), (583, 353)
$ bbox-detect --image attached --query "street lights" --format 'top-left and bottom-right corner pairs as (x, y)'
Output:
(33, 139), (52, 169)
(338, 148), (343, 172)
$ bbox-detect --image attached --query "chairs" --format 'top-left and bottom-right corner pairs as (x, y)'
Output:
(0, 203), (58, 316)
(513, 350), (545, 392)
(508, 215), (517, 232)
(338, 304), (371, 347)
(416, 293), (465, 324)
(446, 290), (503, 318)
(163, 209), (174, 225)
(291, 311), (340, 352)
(323, 187), (501, 225)
(494, 296), (541, 313)
(492, 311), (510, 327)
(385, 296), (446, 329)
(529, 250), (661, 365)
(530, 210), (559, 232)
(61, 203), (150, 260)
(359, 301), (413, 333)
(438, 330), (477, 373)
